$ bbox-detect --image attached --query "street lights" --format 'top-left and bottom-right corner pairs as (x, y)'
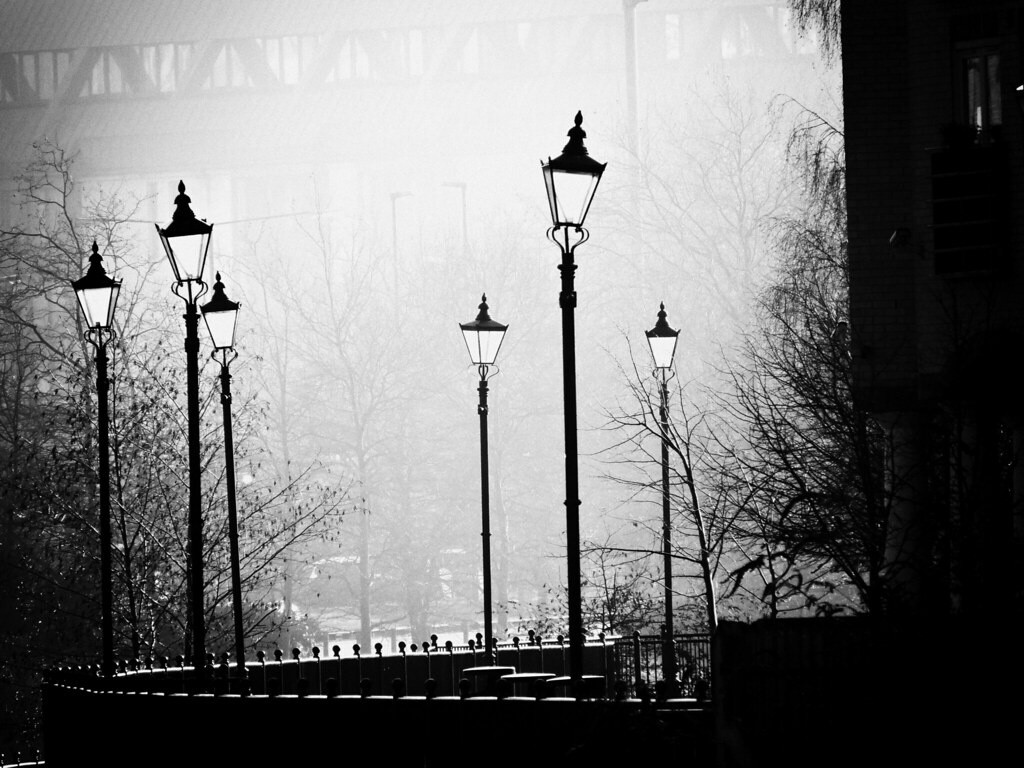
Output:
(199, 272), (250, 688)
(152, 179), (213, 690)
(537, 108), (611, 706)
(70, 239), (124, 689)
(458, 293), (509, 675)
(643, 301), (683, 702)
